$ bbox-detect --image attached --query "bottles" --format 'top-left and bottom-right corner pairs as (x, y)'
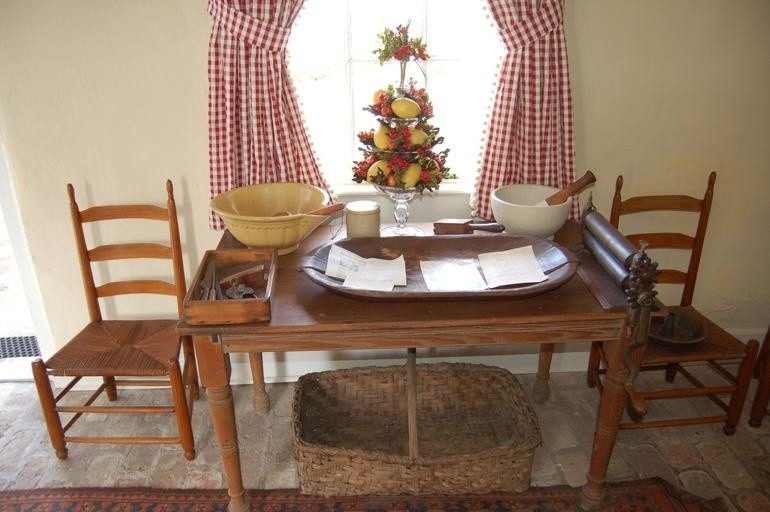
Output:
(343, 199), (381, 238)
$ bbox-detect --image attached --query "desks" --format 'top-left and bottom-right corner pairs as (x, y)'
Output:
(176, 227), (672, 511)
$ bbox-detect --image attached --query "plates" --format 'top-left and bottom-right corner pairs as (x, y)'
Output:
(294, 233), (582, 300)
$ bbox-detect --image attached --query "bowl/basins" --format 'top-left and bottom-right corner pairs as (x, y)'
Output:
(210, 184), (331, 258)
(646, 312), (709, 350)
(490, 182), (570, 238)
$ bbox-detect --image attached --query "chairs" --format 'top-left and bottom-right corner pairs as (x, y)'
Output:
(749, 324), (770, 427)
(579, 169), (760, 437)
(29, 176), (200, 461)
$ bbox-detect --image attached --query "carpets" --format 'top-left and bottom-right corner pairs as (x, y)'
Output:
(1, 475), (720, 511)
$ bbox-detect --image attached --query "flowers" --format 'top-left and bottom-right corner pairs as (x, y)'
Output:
(369, 17), (433, 67)
